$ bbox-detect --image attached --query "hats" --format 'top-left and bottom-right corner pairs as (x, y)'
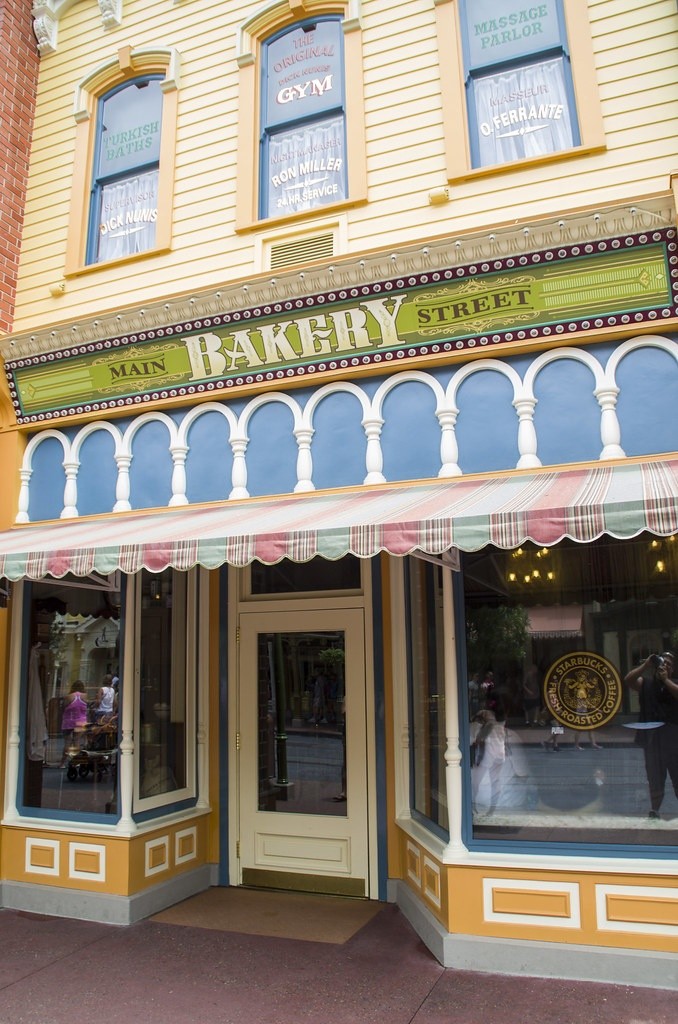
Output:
(110, 677), (119, 685)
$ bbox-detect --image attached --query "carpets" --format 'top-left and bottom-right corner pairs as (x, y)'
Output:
(145, 886), (389, 945)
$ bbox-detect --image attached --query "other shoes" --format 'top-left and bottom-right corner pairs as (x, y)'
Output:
(55, 766), (66, 769)
(331, 793), (346, 802)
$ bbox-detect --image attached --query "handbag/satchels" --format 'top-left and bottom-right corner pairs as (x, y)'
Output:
(88, 707), (96, 718)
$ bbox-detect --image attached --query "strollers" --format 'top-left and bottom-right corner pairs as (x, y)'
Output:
(67, 715), (118, 782)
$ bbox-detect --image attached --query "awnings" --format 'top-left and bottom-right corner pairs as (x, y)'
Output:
(0, 453), (678, 586)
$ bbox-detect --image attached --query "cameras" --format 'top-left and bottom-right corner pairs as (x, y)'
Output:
(649, 655), (664, 668)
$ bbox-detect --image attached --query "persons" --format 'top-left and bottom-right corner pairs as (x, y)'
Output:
(468, 655), (603, 808)
(623, 650), (678, 820)
(333, 696), (346, 800)
(58, 671), (119, 769)
(300, 660), (337, 725)
